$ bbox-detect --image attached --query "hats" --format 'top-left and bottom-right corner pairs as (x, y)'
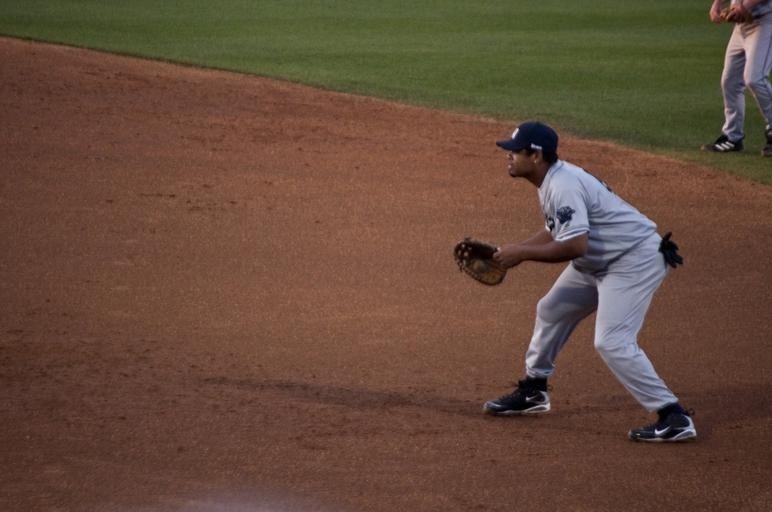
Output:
(496, 120), (559, 154)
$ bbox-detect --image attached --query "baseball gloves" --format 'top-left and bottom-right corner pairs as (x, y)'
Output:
(453, 235), (507, 286)
(719, 0), (753, 24)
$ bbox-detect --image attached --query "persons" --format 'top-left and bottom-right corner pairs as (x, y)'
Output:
(482, 122), (700, 443)
(702, 1), (772, 158)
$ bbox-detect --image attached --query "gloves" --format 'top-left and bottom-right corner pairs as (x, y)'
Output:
(656, 231), (684, 269)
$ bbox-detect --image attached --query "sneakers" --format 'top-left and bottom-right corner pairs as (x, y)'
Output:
(627, 409), (696, 442)
(760, 124), (772, 156)
(481, 376), (552, 415)
(700, 133), (746, 153)
